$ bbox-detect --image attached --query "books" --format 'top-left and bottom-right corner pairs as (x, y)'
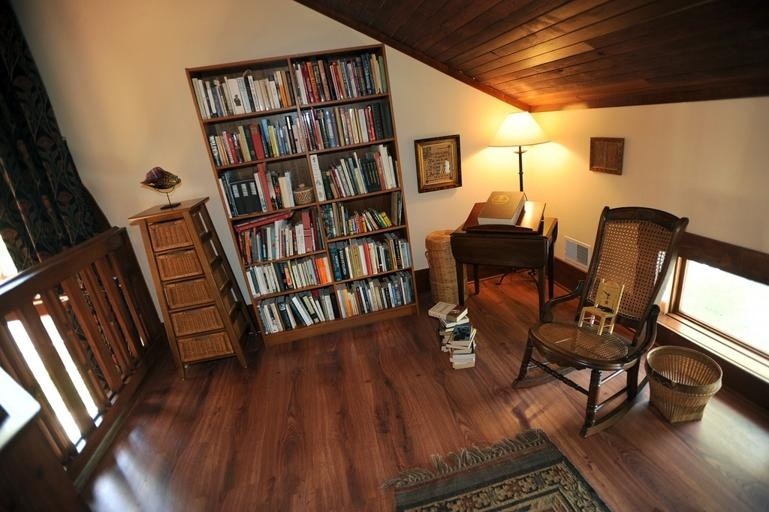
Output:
(477, 190), (527, 225)
(216, 161), (325, 265)
(290, 54), (394, 153)
(426, 299), (477, 371)
(243, 233), (412, 335)
(191, 71), (304, 165)
(309, 143), (403, 239)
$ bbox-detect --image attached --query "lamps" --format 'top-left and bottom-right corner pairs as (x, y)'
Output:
(485, 110), (553, 288)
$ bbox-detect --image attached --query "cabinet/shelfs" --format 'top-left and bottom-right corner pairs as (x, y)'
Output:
(127, 198), (258, 378)
(184, 44), (422, 354)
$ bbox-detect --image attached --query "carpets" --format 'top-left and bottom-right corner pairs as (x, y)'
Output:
(377, 427), (613, 511)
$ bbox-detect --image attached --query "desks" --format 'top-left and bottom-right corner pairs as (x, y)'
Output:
(448, 218), (558, 322)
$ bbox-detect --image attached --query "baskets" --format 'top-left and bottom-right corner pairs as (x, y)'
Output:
(644, 345), (724, 425)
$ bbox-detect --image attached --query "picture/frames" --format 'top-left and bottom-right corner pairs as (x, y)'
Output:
(413, 133), (463, 195)
(588, 135), (626, 177)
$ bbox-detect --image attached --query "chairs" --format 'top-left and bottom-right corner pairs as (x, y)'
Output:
(511, 206), (691, 440)
